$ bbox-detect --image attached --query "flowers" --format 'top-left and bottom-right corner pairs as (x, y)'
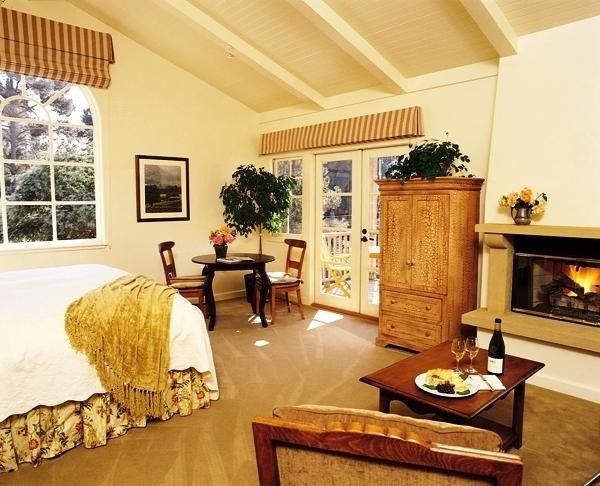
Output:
(498, 187), (548, 217)
(208, 224), (237, 245)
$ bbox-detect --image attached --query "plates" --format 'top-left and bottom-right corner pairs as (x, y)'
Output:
(415, 371), (481, 398)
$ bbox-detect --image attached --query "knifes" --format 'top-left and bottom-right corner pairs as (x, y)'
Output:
(477, 372), (495, 392)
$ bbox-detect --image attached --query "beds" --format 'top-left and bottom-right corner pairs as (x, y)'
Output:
(0, 262), (220, 474)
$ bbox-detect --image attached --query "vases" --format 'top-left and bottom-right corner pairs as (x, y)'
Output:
(511, 205), (531, 225)
(214, 244), (228, 260)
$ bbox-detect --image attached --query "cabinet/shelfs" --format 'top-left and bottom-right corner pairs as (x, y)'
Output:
(371, 178), (485, 352)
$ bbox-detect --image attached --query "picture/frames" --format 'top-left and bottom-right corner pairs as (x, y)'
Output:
(135, 154), (190, 222)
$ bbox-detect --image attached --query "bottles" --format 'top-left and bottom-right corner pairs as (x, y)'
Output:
(487, 318), (505, 376)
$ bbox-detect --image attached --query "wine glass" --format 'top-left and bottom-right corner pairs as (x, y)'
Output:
(465, 336), (480, 374)
(450, 337), (466, 375)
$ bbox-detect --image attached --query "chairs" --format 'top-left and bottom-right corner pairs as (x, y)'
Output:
(250, 404), (524, 486)
(320, 235), (352, 298)
(159, 241), (210, 320)
(256, 239), (307, 324)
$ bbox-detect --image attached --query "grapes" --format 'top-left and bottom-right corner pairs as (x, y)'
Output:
(437, 383), (455, 394)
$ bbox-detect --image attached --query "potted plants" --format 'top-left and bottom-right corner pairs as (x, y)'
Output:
(386, 133), (476, 184)
(218, 162), (299, 303)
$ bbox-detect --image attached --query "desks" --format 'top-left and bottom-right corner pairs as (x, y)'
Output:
(369, 246), (379, 259)
(191, 252), (276, 331)
